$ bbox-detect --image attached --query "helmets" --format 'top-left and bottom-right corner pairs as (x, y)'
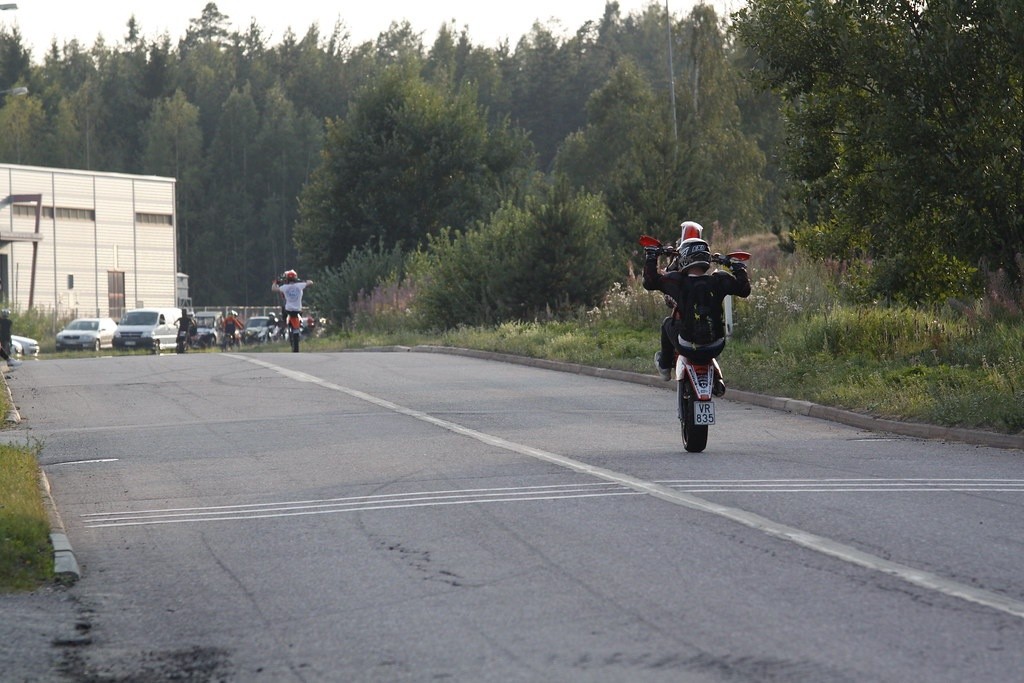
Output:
(228, 310), (238, 317)
(676, 238), (711, 273)
(284, 270), (297, 284)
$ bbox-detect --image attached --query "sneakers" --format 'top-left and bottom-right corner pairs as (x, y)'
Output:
(714, 379), (726, 397)
(654, 351), (671, 381)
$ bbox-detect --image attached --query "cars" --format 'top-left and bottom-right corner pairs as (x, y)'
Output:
(244, 317), (271, 341)
(55, 318), (118, 351)
(11, 334), (40, 358)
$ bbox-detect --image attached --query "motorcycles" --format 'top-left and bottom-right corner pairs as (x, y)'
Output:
(298, 316), (328, 339)
(175, 324), (195, 354)
(239, 324), (289, 346)
(272, 269), (305, 353)
(639, 220), (751, 453)
(221, 328), (242, 353)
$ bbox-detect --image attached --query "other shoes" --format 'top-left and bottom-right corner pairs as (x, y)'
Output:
(7, 357), (22, 367)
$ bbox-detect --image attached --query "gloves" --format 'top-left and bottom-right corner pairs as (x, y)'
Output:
(728, 257), (748, 275)
(644, 246), (659, 260)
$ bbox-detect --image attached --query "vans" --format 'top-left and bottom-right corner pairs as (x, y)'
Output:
(194, 311), (223, 346)
(111, 308), (183, 355)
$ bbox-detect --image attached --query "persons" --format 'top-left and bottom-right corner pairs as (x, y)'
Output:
(174, 312), (196, 330)
(224, 310), (243, 343)
(642, 238), (752, 382)
(266, 312), (282, 327)
(272, 269), (313, 321)
